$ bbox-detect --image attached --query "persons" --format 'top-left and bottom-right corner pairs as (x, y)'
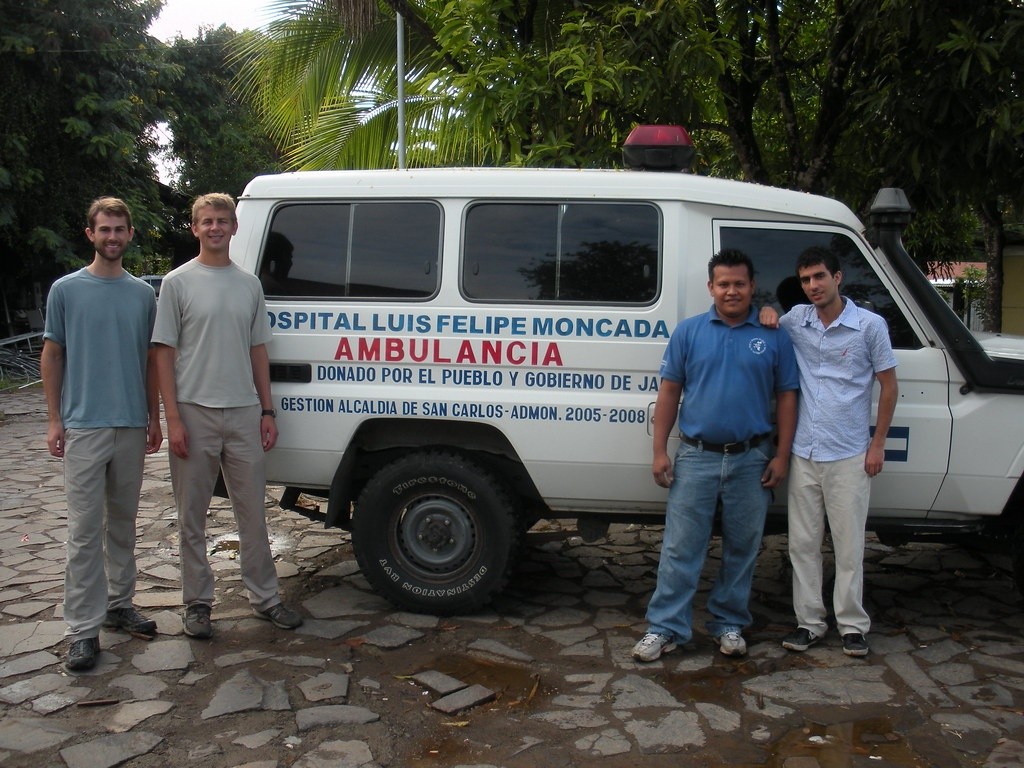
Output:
(150, 193), (304, 639)
(759, 248), (898, 658)
(40, 196), (157, 671)
(629, 250), (800, 663)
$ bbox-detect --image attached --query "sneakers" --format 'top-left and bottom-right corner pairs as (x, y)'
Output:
(781, 627), (820, 651)
(631, 633), (678, 660)
(102, 606), (158, 631)
(252, 602), (302, 628)
(182, 603), (212, 637)
(712, 632), (746, 655)
(65, 636), (100, 671)
(841, 632), (869, 656)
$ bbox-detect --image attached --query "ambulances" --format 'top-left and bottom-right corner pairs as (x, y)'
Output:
(209, 125), (1024, 617)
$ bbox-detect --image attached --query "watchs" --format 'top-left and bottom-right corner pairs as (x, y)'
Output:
(261, 408), (278, 418)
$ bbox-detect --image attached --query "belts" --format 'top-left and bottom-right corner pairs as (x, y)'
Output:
(678, 431), (770, 455)
